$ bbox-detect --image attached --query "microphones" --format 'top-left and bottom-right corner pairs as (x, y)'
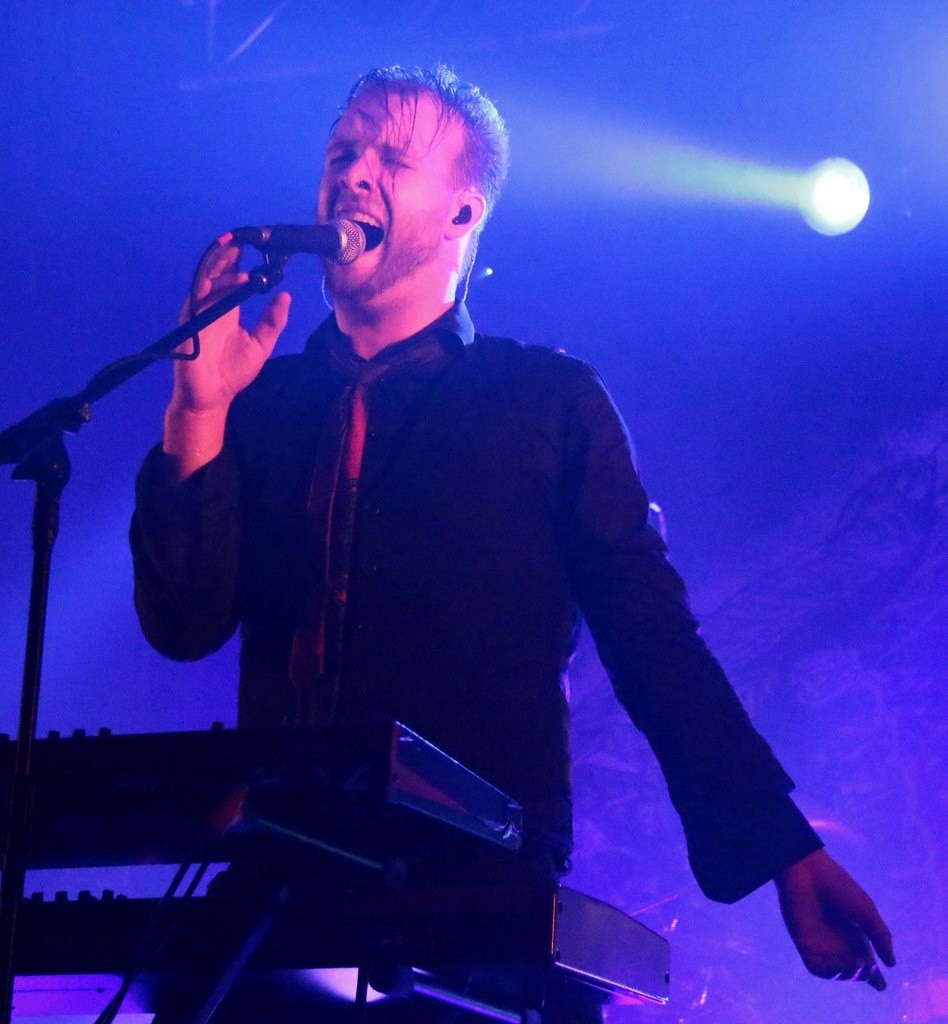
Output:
(231, 221), (364, 265)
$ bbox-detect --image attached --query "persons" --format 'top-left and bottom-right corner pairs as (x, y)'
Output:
(127, 58), (903, 1024)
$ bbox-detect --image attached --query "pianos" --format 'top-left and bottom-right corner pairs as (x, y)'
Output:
(0, 716), (525, 1024)
